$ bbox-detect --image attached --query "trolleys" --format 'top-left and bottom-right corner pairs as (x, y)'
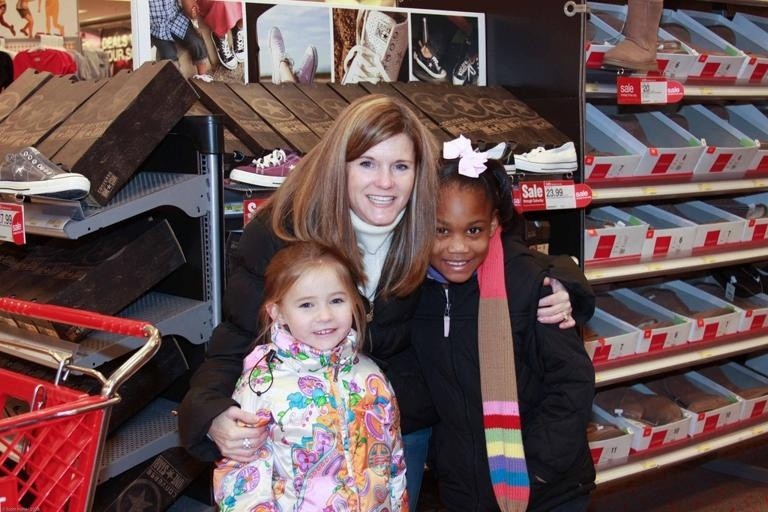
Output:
(0, 297), (162, 512)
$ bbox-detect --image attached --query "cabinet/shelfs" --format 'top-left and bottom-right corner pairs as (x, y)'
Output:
(0, 115), (221, 512)
(396, 1), (768, 486)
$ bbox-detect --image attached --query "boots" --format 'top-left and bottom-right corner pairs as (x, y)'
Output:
(585, 2), (766, 451)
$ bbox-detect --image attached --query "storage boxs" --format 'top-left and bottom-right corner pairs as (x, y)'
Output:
(584, 96), (768, 187)
(588, 349), (767, 471)
(580, 0), (768, 86)
(583, 190), (768, 262)
(583, 256), (767, 366)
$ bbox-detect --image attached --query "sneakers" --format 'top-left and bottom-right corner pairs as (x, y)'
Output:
(293, 45), (319, 84)
(268, 25), (291, 85)
(452, 42), (480, 86)
(340, 9), (408, 85)
(209, 28), (237, 72)
(227, 26), (250, 62)
(228, 150), (305, 187)
(0, 142), (93, 204)
(412, 44), (447, 79)
(516, 142), (581, 174)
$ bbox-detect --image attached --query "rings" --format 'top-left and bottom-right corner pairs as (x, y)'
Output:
(563, 310), (570, 320)
(243, 437), (252, 449)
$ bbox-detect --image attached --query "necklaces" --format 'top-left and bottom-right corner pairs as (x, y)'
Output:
(357, 232), (394, 257)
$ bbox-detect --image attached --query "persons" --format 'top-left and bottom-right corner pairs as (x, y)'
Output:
(38, 0), (64, 35)
(183, 0), (245, 70)
(211, 241), (412, 511)
(412, 15), (447, 79)
(0, 0), (16, 35)
(17, 0), (34, 38)
(365, 132), (597, 511)
(451, 17), (479, 85)
(267, 26), (318, 83)
(148, 1), (213, 81)
(178, 90), (601, 512)
